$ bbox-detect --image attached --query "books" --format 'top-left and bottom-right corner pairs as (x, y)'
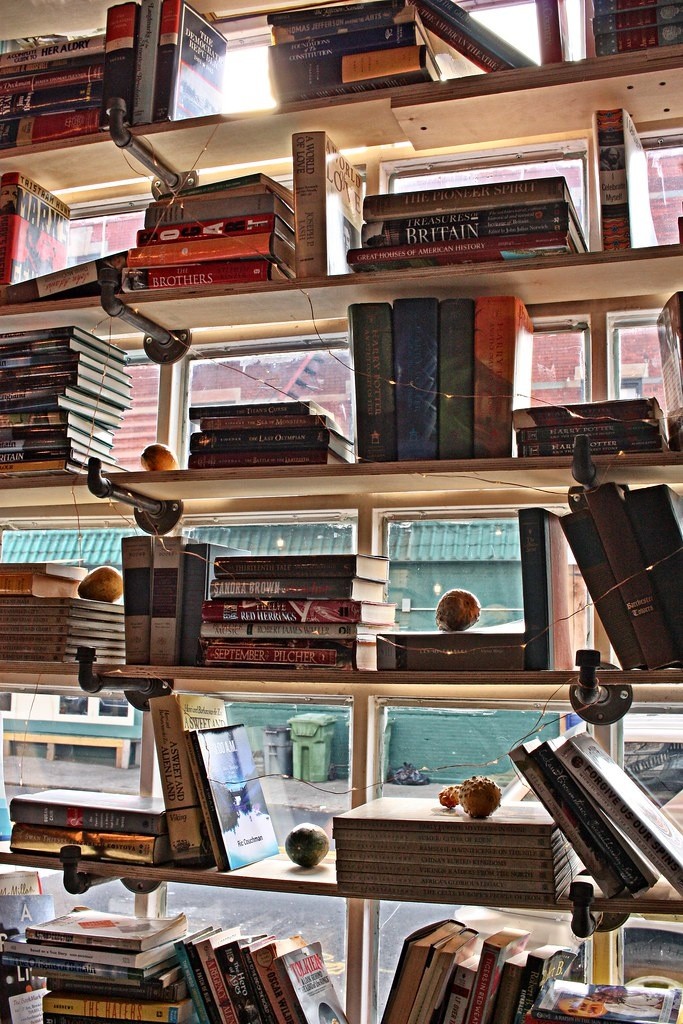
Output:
(186, 291), (683, 469)
(0, 108), (660, 292)
(0, 483), (683, 671)
(0, 695), (683, 1024)
(0, 0), (683, 150)
(0, 326), (134, 477)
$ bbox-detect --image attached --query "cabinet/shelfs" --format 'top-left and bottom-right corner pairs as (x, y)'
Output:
(0, 0), (683, 1024)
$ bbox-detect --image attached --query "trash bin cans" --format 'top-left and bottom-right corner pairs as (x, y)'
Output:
(345, 718), (396, 782)
(262, 724), (294, 779)
(287, 714), (336, 783)
